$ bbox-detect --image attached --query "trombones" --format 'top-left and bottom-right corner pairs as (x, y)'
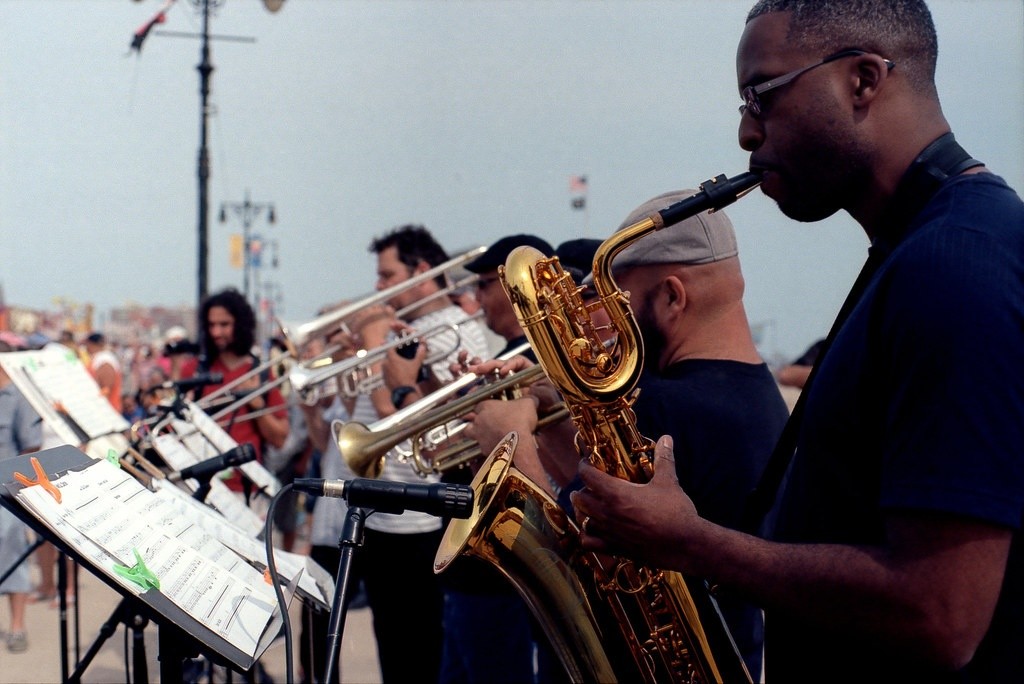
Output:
(130, 359), (332, 448)
(151, 244), (490, 449)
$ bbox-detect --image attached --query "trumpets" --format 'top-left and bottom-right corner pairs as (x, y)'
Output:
(288, 308), (485, 407)
(330, 341), (530, 453)
(339, 337), (617, 476)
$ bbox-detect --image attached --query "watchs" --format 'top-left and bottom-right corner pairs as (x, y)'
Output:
(390, 385), (417, 408)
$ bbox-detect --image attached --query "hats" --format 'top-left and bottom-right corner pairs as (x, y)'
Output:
(582, 188), (740, 285)
(463, 234), (558, 273)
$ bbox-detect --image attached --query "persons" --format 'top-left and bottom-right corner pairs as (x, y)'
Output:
(0, 365), (40, 655)
(172, 284), (289, 520)
(266, 222), (604, 680)
(569, 0), (1024, 684)
(1, 329), (201, 425)
(26, 420), (79, 609)
(778, 338), (827, 388)
(470, 188), (792, 684)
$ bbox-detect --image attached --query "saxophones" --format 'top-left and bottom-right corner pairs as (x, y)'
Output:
(429, 167), (765, 684)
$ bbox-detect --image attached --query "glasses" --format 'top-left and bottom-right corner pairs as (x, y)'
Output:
(476, 275), (497, 290)
(739, 48), (896, 115)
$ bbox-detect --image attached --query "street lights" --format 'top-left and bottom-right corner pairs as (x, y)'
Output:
(125, 0), (287, 372)
(217, 186), (281, 301)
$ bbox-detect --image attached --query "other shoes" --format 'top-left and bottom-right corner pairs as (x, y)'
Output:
(50, 594), (74, 611)
(8, 634), (27, 652)
(25, 592), (56, 604)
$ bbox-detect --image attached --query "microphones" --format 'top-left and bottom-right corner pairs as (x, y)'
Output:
(160, 373), (224, 389)
(167, 444), (257, 483)
(290, 477), (474, 520)
